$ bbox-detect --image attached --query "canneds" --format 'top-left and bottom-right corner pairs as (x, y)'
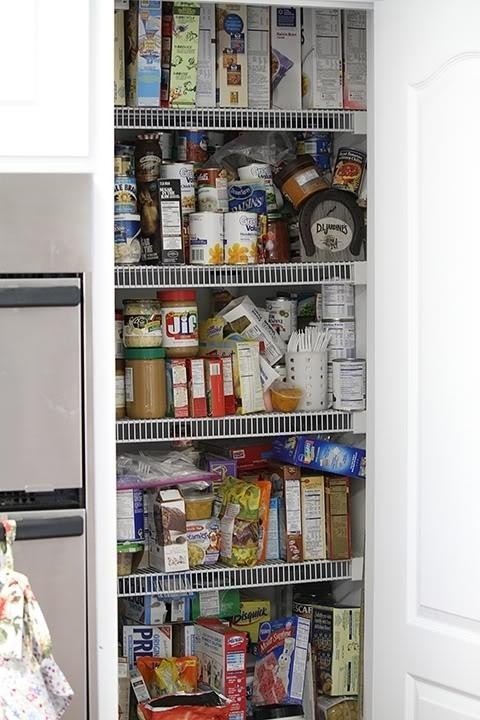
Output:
(115, 145), (135, 175)
(262, 212), (291, 262)
(115, 174), (138, 216)
(332, 148), (366, 194)
(180, 122), (208, 164)
(122, 297), (164, 349)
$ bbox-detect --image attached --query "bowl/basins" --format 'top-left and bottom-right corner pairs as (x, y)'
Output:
(270, 380), (306, 414)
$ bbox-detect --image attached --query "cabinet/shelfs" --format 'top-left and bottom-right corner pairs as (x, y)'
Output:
(98, 0), (375, 720)
